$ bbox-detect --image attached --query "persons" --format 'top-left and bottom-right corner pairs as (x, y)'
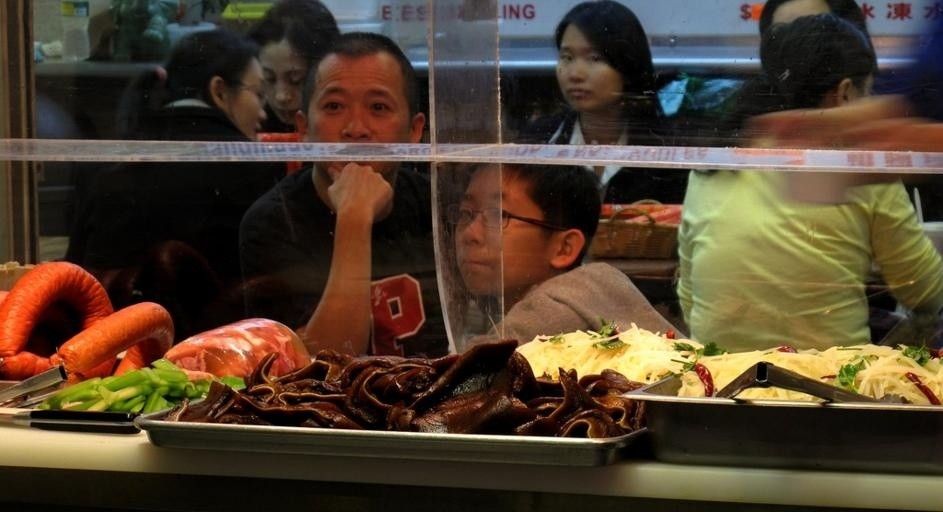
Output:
(511, 0), (682, 205)
(237, 30), (451, 363)
(244, 2), (341, 144)
(671, 13), (942, 358)
(67, 30), (281, 345)
(757, 0), (875, 47)
(727, 3), (943, 199)
(448, 147), (692, 359)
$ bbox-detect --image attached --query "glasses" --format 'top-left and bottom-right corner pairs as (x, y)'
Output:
(442, 200), (567, 239)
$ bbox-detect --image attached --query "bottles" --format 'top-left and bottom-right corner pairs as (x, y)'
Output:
(34, 38), (63, 62)
(59, 0), (92, 62)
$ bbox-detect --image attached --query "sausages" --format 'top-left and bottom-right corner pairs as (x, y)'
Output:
(0, 261), (174, 385)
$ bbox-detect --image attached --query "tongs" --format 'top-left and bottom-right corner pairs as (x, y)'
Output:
(711, 360), (912, 404)
